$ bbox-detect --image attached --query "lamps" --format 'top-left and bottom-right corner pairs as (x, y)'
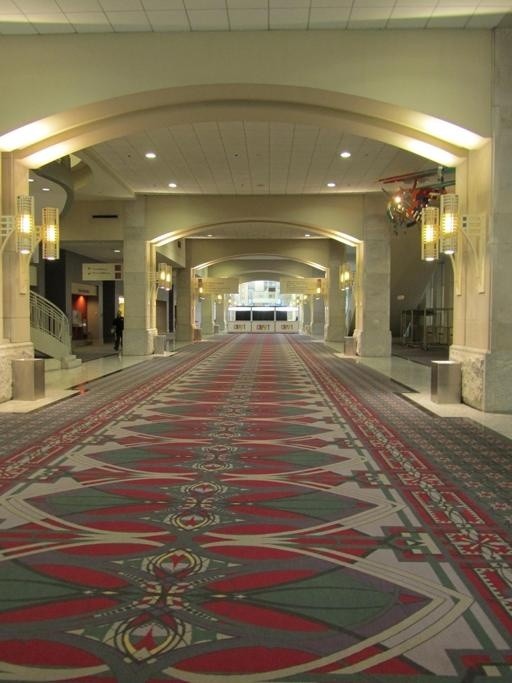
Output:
(338, 262), (360, 307)
(20, 205), (61, 266)
(438, 193), (487, 295)
(0, 196), (34, 260)
(150, 261), (173, 304)
(419, 205), (463, 295)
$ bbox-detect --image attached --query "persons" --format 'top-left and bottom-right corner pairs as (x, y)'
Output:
(110, 310), (124, 351)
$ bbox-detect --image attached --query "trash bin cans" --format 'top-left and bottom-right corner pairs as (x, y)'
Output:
(194, 329), (202, 340)
(154, 335), (165, 354)
(214, 326), (220, 334)
(305, 325), (311, 335)
(431, 360), (462, 404)
(344, 337), (357, 355)
(10, 358), (45, 401)
